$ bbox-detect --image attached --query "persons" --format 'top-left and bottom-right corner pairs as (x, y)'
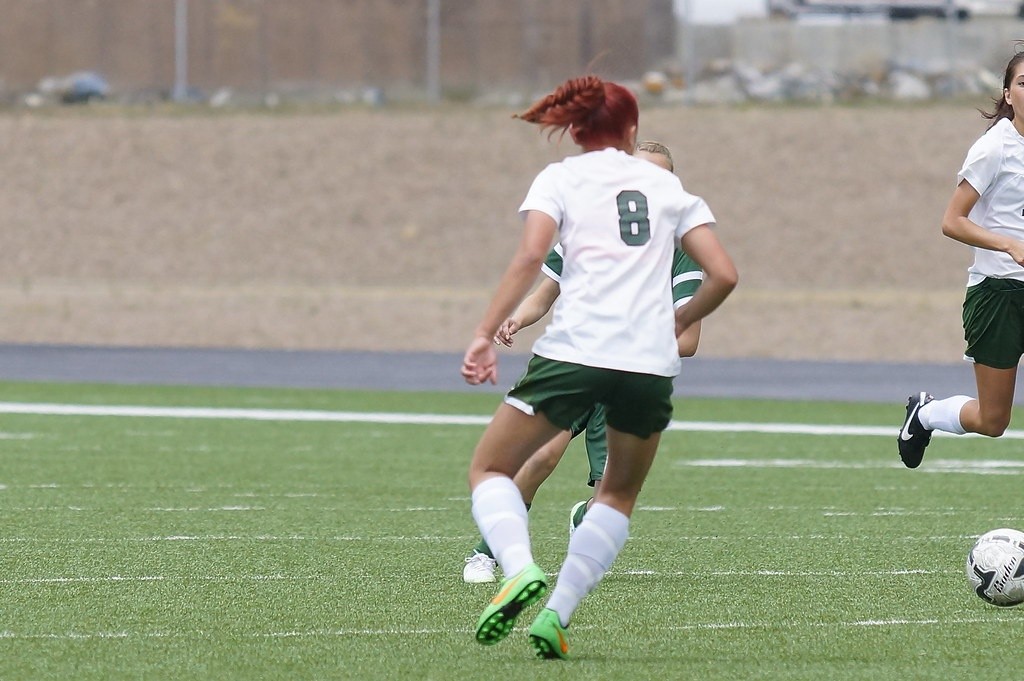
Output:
(460, 76), (738, 660)
(464, 141), (704, 585)
(898, 51), (1024, 469)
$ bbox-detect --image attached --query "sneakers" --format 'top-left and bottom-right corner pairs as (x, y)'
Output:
(528, 605), (572, 661)
(474, 567), (547, 646)
(568, 498), (594, 542)
(463, 549), (496, 584)
(897, 392), (934, 469)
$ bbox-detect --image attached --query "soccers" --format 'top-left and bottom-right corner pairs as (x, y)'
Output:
(963, 527), (1024, 608)
